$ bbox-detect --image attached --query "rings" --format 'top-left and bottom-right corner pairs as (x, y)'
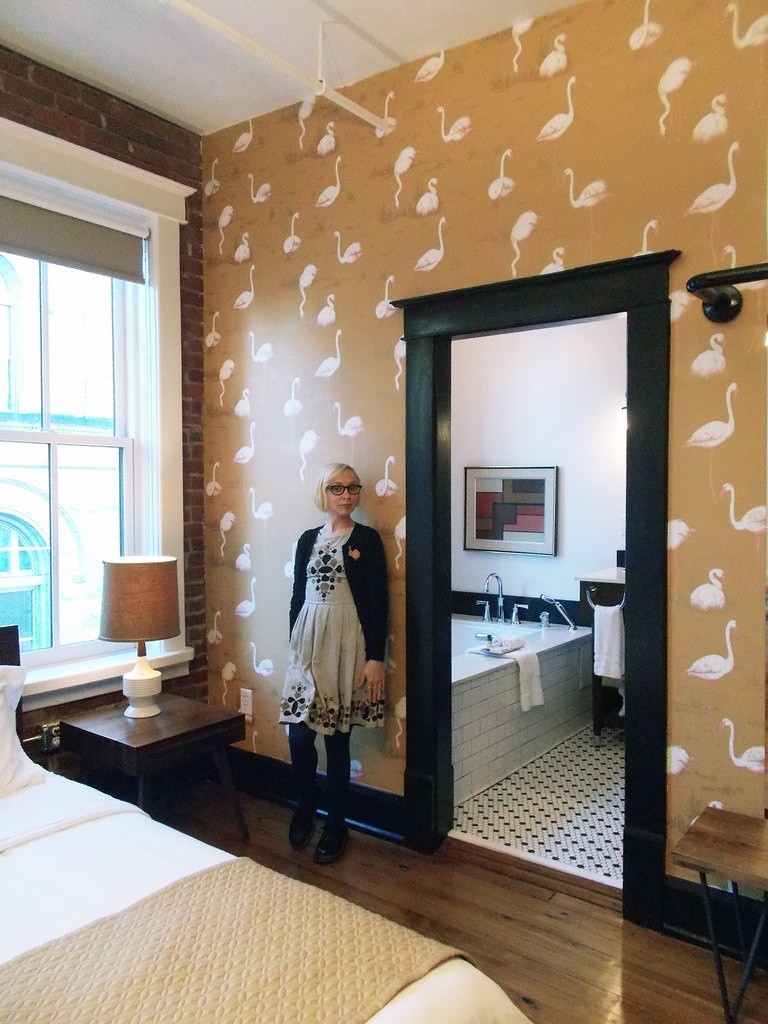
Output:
(367, 686), (373, 689)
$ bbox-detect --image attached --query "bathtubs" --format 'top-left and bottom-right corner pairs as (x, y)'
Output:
(452, 609), (594, 808)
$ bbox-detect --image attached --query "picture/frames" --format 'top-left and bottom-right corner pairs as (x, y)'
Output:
(462, 465), (559, 559)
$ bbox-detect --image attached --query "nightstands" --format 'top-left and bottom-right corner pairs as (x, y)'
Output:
(58, 691), (253, 846)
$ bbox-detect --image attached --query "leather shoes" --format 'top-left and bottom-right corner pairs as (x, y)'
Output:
(313, 820), (349, 863)
(288, 787), (323, 848)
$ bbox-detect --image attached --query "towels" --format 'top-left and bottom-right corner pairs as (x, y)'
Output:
(594, 603), (625, 680)
(464, 633), (546, 714)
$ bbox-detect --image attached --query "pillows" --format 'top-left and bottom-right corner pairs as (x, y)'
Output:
(0, 664), (48, 800)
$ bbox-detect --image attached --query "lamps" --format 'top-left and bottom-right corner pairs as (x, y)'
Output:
(95, 554), (182, 721)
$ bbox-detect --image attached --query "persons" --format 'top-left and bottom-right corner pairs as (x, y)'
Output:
(274, 464), (392, 865)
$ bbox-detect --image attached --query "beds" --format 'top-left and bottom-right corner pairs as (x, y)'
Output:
(0, 759), (536, 1024)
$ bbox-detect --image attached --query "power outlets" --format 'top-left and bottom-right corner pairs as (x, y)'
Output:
(239, 688), (253, 716)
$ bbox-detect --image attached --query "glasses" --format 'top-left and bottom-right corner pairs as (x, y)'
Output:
(327, 484), (362, 495)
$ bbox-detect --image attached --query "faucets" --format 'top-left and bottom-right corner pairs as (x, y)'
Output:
(483, 572), (509, 624)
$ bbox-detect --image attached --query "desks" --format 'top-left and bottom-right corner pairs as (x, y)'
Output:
(670, 805), (768, 1024)
(572, 567), (626, 751)
